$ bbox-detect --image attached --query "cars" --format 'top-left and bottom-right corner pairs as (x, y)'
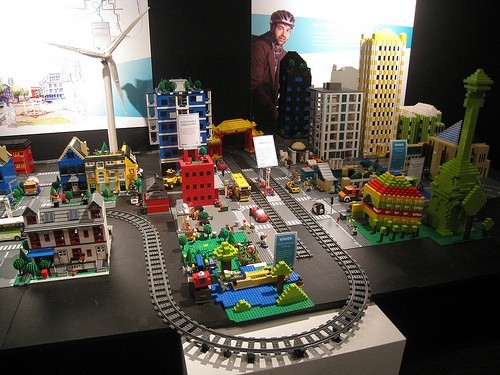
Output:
(250, 206), (269, 223)
(313, 203), (325, 215)
(286, 181), (301, 195)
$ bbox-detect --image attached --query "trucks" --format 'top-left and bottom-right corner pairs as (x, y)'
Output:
(338, 186), (362, 203)
(25, 177), (39, 196)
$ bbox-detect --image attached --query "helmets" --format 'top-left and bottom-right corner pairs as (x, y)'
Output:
(270, 10), (295, 29)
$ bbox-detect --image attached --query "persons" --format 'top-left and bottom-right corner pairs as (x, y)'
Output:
(252, 10), (295, 139)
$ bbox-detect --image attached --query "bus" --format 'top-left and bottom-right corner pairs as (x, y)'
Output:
(231, 172), (252, 202)
